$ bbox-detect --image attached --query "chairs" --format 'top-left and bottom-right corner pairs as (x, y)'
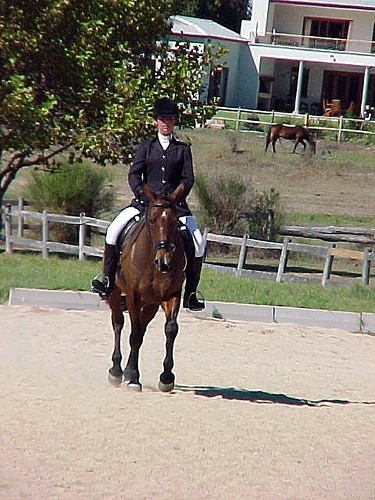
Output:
(322, 97), (354, 116)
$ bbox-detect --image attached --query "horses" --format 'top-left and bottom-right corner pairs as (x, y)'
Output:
(100, 183), (186, 391)
(264, 124), (317, 155)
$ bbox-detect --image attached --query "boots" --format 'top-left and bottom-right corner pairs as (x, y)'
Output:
(92, 241), (120, 300)
(183, 256), (205, 308)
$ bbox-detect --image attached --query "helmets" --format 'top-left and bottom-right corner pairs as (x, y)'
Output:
(153, 98), (178, 117)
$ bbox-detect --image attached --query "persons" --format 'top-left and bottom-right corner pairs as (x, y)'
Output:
(92, 98), (208, 311)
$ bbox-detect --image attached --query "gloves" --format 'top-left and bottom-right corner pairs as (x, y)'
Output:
(139, 192), (149, 207)
